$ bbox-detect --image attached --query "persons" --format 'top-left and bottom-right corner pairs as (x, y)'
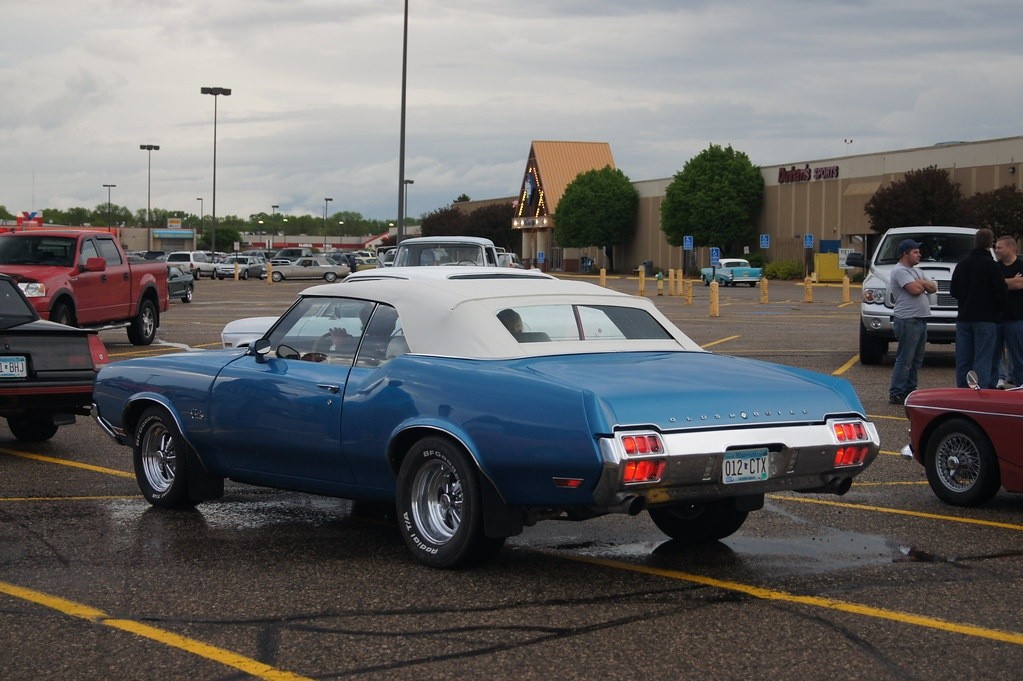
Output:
(349, 253), (357, 274)
(889, 239), (938, 405)
(329, 302), (399, 349)
(995, 236), (1023, 388)
(950, 228), (1008, 389)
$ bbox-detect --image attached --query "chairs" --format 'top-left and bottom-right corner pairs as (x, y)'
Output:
(385, 337), (410, 360)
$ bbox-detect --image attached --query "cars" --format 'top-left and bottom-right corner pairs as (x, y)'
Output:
(494, 246), (526, 269)
(123, 249), (168, 261)
(206, 245), (398, 274)
(220, 265), (626, 352)
(394, 235), (499, 271)
(169, 267), (197, 300)
(269, 256), (354, 283)
(216, 255), (264, 279)
(0, 273), (110, 443)
(89, 280), (883, 569)
(902, 371), (1023, 508)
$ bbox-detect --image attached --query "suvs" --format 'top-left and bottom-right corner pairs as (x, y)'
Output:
(844, 225), (1006, 359)
(165, 249), (218, 280)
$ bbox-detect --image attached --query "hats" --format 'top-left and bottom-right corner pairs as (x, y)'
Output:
(897, 239), (923, 260)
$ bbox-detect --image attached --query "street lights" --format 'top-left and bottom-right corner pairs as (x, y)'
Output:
(402, 179), (415, 236)
(102, 184), (117, 234)
(195, 197), (208, 240)
(271, 204), (280, 247)
(139, 143), (161, 255)
(201, 86), (233, 261)
(323, 196), (337, 252)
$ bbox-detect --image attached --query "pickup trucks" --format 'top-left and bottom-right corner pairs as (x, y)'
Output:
(700, 259), (769, 286)
(0, 228), (173, 346)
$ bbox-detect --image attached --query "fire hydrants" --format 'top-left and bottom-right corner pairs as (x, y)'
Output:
(655, 272), (679, 300)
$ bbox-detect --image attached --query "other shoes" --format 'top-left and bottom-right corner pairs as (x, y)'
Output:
(996, 379), (1023, 391)
(889, 393), (906, 405)
